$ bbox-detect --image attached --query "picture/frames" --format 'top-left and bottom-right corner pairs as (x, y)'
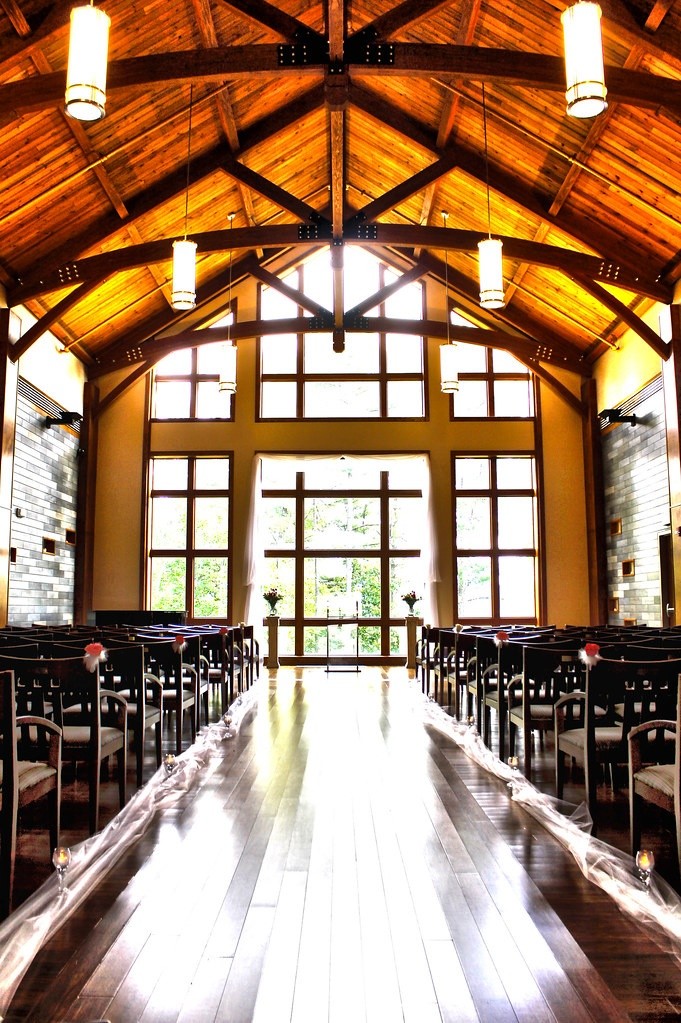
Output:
(622, 558), (634, 577)
(65, 528), (78, 545)
(624, 618), (638, 626)
(609, 517), (623, 536)
(42, 536), (57, 556)
(607, 597), (619, 613)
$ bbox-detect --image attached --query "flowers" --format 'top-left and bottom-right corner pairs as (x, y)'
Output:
(263, 588), (284, 605)
(401, 590), (422, 606)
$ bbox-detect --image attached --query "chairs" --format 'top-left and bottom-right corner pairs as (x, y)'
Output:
(0, 620), (261, 924)
(419, 623), (679, 855)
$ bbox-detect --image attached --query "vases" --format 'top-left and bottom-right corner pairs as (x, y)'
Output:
(270, 607), (277, 616)
(408, 606), (415, 613)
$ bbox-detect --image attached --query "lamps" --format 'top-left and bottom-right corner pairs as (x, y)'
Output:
(65, 0), (110, 121)
(439, 208), (461, 394)
(561, 0), (610, 120)
(172, 83), (198, 312)
(598, 407), (636, 426)
(477, 83), (507, 308)
(43, 411), (83, 428)
(220, 213), (239, 396)
